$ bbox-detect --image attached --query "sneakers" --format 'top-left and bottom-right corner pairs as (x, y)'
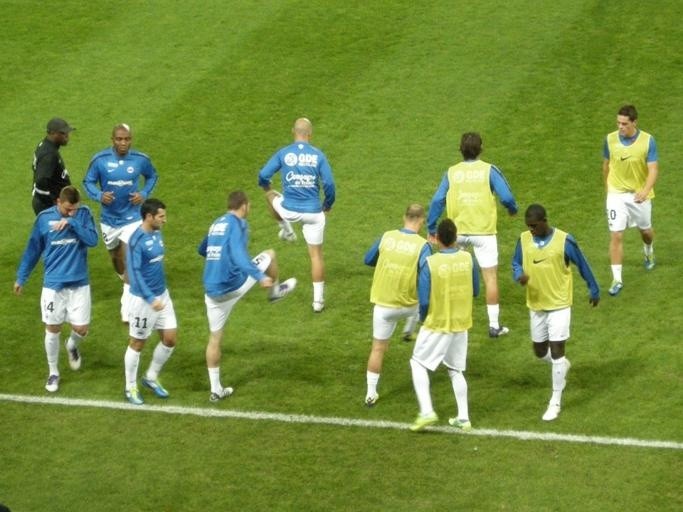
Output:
(562, 359), (571, 389)
(609, 279), (624, 296)
(121, 294), (131, 322)
(489, 325), (509, 337)
(644, 254), (655, 270)
(365, 393), (471, 432)
(44, 374), (60, 392)
(542, 405), (561, 421)
(268, 278), (296, 302)
(210, 387), (234, 402)
(278, 229), (297, 241)
(124, 386), (144, 404)
(402, 331), (416, 341)
(311, 299), (325, 311)
(65, 337), (82, 370)
(142, 376), (170, 398)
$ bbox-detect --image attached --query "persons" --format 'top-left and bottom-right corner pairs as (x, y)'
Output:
(426, 132), (519, 338)
(258, 118), (335, 314)
(82, 123), (159, 324)
(198, 191), (297, 403)
(407, 219), (479, 433)
(14, 186), (99, 393)
(512, 204), (600, 421)
(124, 198), (178, 405)
(32, 118), (76, 216)
(603, 106), (658, 297)
(364, 204), (433, 406)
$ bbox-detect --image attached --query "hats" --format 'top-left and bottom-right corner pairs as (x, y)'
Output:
(47, 117), (76, 134)
